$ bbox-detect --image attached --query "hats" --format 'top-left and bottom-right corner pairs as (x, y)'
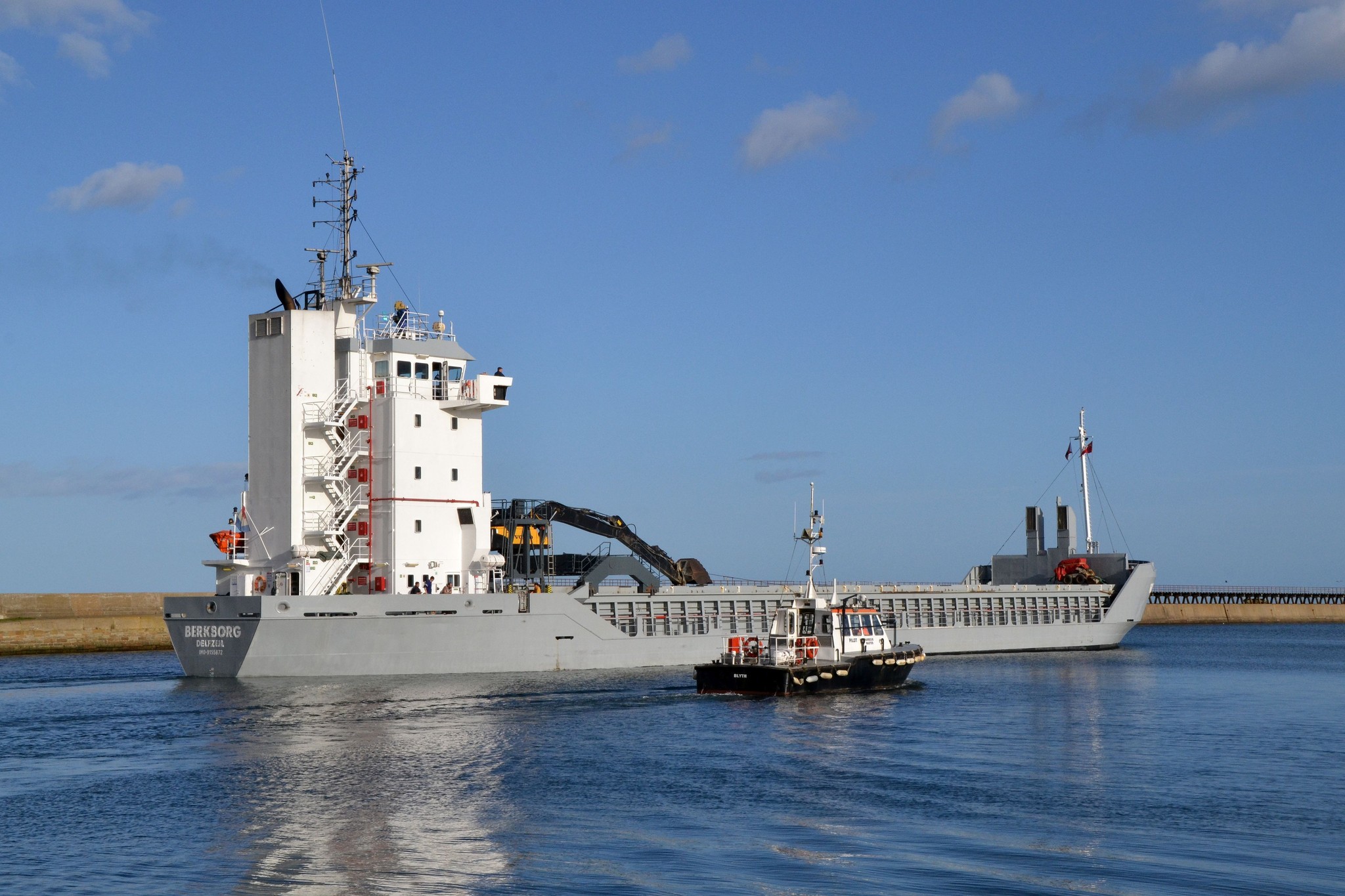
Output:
(431, 576), (434, 579)
(534, 583), (539, 586)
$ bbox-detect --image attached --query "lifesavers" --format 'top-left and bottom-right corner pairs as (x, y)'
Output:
(254, 576), (266, 591)
(807, 638), (818, 658)
(744, 637), (763, 657)
(738, 637), (746, 651)
(462, 382), (477, 396)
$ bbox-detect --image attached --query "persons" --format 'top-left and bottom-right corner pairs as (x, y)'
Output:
(534, 583), (541, 593)
(857, 622), (870, 635)
(410, 582), (422, 615)
(494, 367), (505, 377)
(440, 583), (452, 615)
(423, 576), (436, 614)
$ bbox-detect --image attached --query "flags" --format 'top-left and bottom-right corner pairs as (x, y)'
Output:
(235, 506), (251, 532)
(1078, 441), (1093, 457)
(1065, 442), (1073, 460)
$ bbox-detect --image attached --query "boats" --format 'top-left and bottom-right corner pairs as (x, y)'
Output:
(692, 483), (926, 693)
(159, 12), (1159, 687)
(208, 530), (250, 557)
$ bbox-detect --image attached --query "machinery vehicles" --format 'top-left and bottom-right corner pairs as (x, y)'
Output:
(489, 496), (717, 587)
